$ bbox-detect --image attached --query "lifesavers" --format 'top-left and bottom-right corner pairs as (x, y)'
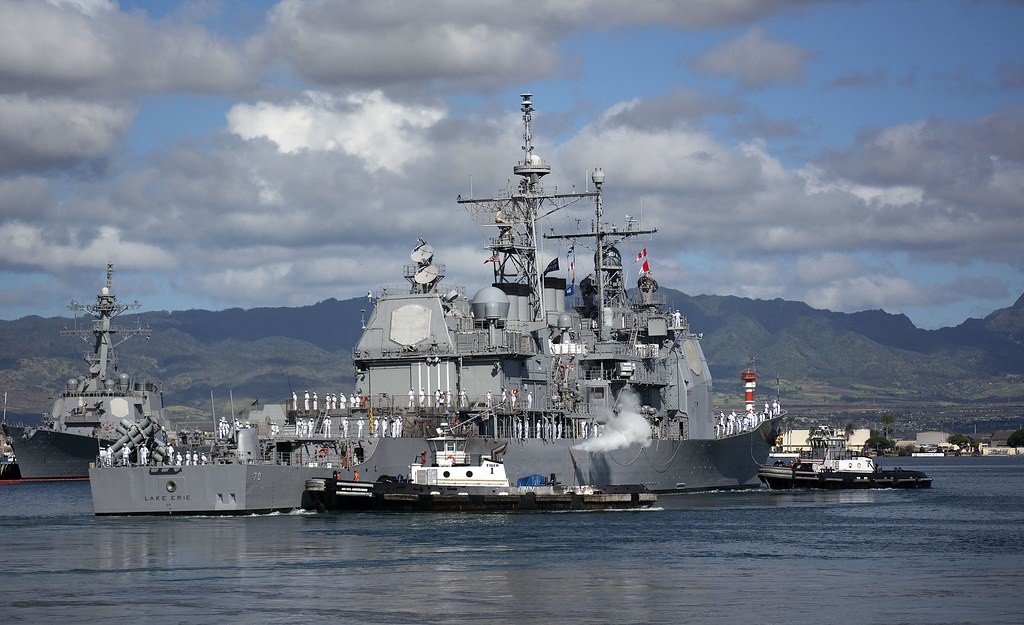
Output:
(447, 456), (455, 465)
(638, 276), (658, 292)
(318, 448), (327, 458)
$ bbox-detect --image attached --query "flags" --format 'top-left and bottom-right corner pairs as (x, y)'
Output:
(568, 245), (574, 253)
(568, 262), (575, 271)
(484, 253), (499, 264)
(635, 248), (647, 262)
(640, 260), (649, 274)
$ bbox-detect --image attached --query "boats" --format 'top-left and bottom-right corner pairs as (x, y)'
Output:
(300, 423), (658, 513)
(1, 263), (211, 478)
(89, 93), (788, 516)
(769, 446), (804, 458)
(758, 426), (934, 490)
(912, 446), (945, 458)
(988, 450), (1008, 456)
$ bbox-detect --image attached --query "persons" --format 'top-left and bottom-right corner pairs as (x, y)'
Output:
(99, 444), (149, 466)
(165, 443), (206, 465)
(326, 393), (360, 409)
(408, 388), (466, 407)
(584, 422), (599, 439)
(721, 400), (781, 437)
(517, 418), (563, 439)
(374, 416), (402, 437)
(297, 416), (331, 438)
(292, 391), (318, 410)
(486, 389), (532, 409)
(342, 416), (364, 438)
(219, 418), (250, 438)
